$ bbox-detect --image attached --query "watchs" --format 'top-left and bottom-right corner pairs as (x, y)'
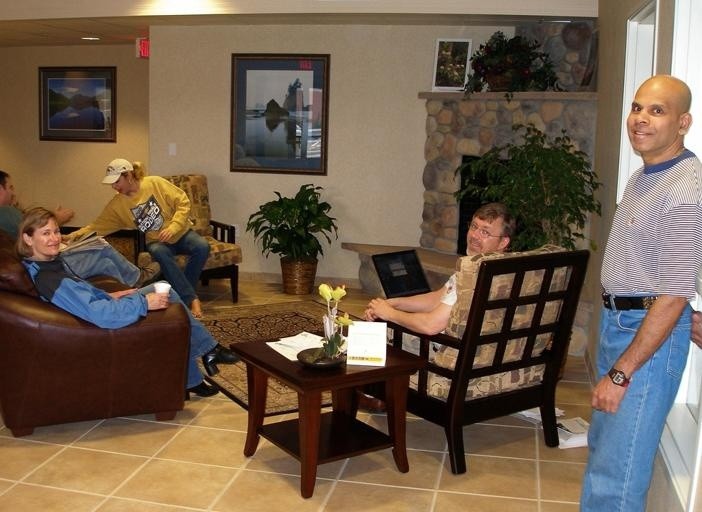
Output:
(607, 367), (631, 387)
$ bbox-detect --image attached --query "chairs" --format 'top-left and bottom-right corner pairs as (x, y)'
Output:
(376, 249), (589, 475)
(136, 175), (242, 304)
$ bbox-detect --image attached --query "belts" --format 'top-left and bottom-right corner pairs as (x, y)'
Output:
(600, 291), (658, 311)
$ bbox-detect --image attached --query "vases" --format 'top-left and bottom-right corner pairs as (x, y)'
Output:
(484, 69), (518, 92)
(296, 347), (347, 377)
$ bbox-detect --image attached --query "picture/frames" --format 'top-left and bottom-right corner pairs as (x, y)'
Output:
(230, 52), (330, 176)
(38, 66), (116, 143)
(432, 38), (472, 92)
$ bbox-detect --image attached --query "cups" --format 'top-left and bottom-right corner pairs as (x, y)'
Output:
(154, 283), (172, 293)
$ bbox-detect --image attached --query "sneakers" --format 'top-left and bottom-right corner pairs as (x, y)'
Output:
(133, 261), (161, 290)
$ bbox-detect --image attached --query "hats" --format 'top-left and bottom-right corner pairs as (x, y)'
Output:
(101, 158), (135, 184)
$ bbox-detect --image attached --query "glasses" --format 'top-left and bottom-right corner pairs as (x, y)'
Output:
(467, 221), (505, 239)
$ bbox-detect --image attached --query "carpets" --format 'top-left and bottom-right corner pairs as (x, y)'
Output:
(190, 300), (386, 416)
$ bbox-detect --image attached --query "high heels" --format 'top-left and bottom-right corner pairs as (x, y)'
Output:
(202, 343), (240, 377)
(184, 380), (218, 402)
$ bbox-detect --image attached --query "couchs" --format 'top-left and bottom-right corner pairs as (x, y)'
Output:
(0, 248), (188, 439)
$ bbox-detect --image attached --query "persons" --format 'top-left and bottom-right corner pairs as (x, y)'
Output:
(363, 203), (516, 362)
(0, 170), (162, 290)
(579, 75), (702, 508)
(62, 158), (211, 320)
(16, 208), (240, 398)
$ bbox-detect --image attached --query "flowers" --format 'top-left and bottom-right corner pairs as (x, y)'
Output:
(463, 30), (568, 100)
(316, 284), (352, 361)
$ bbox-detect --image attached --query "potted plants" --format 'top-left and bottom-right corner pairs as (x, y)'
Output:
(246, 185), (337, 294)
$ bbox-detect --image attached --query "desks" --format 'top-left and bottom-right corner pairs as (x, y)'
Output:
(230, 326), (427, 499)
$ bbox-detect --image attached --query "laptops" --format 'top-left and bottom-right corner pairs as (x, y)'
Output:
(371, 249), (432, 299)
